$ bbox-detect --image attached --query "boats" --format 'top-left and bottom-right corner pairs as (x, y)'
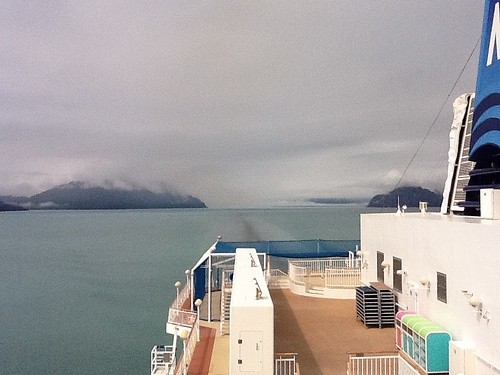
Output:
(152, 0), (500, 375)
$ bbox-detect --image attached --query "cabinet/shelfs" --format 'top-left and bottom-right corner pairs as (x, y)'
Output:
(395, 310), (453, 374)
(355, 282), (396, 329)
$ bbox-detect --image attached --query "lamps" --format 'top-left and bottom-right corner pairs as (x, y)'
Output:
(356, 251), (368, 269)
(460, 289), (480, 308)
(381, 261), (391, 276)
(419, 278), (430, 289)
(396, 270), (407, 284)
